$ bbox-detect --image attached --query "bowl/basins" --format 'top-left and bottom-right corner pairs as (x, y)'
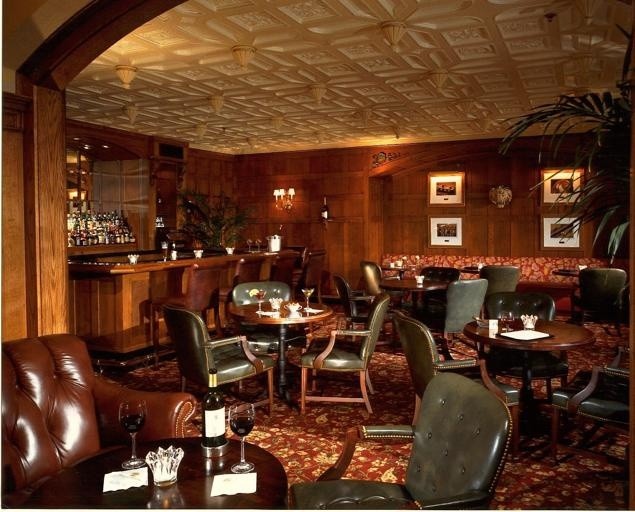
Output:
(476, 320), (488, 328)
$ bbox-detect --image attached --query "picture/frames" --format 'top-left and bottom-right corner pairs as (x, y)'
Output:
(427, 171), (465, 208)
(540, 167), (584, 207)
(428, 214), (465, 249)
(540, 212), (584, 251)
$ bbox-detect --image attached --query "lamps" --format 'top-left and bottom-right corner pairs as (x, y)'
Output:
(273, 188), (296, 210)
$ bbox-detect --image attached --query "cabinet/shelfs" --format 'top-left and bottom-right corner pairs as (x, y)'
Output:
(64, 116), (191, 261)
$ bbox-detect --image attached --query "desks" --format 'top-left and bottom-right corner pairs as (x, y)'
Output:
(21, 436), (288, 508)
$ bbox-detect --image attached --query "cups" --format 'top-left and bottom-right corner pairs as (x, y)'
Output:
(284, 312), (303, 318)
(578, 264), (588, 270)
(520, 313), (538, 330)
(225, 247), (235, 255)
(396, 261), (402, 267)
(489, 319), (499, 338)
(193, 250), (204, 260)
(477, 263), (486, 271)
(145, 445), (185, 488)
(389, 263), (394, 268)
(415, 275), (425, 289)
(284, 302), (302, 312)
(268, 297), (284, 310)
(127, 254), (140, 265)
(161, 241), (170, 262)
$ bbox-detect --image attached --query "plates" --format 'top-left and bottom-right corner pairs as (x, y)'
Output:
(495, 328), (555, 341)
(559, 268), (578, 272)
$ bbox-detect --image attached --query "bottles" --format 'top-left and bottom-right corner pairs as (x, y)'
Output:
(159, 216), (164, 226)
(201, 367), (228, 458)
(320, 196), (328, 219)
(415, 256), (419, 266)
(169, 240), (177, 261)
(155, 216), (160, 226)
(402, 256), (407, 267)
(157, 187), (162, 205)
(67, 210), (136, 248)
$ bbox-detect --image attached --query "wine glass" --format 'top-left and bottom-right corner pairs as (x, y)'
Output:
(118, 398), (147, 469)
(247, 238), (253, 252)
(253, 289), (267, 314)
(228, 403), (255, 473)
(255, 239), (262, 253)
(498, 311), (516, 332)
(301, 287), (315, 308)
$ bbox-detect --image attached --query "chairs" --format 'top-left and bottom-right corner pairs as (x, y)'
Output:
(1, 335), (198, 508)
